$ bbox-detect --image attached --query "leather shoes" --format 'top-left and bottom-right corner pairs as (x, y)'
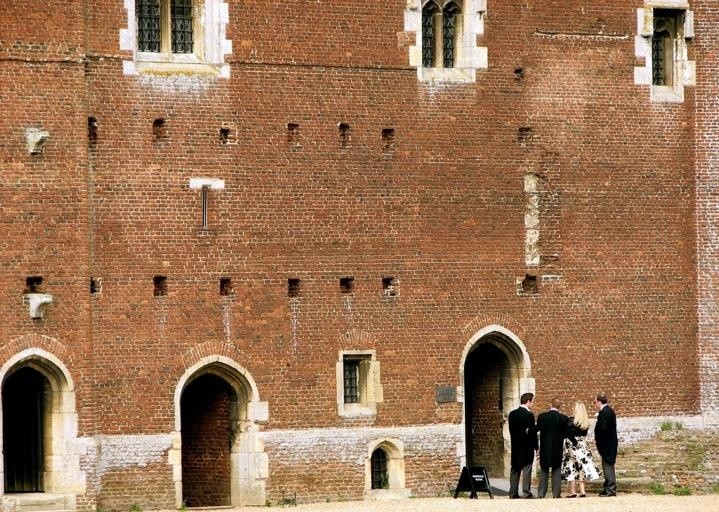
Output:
(599, 489), (616, 497)
(567, 494), (586, 497)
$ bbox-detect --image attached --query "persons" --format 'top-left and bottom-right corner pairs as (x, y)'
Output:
(525, 397), (579, 498)
(594, 395), (618, 497)
(560, 401), (599, 497)
(508, 393), (539, 498)
